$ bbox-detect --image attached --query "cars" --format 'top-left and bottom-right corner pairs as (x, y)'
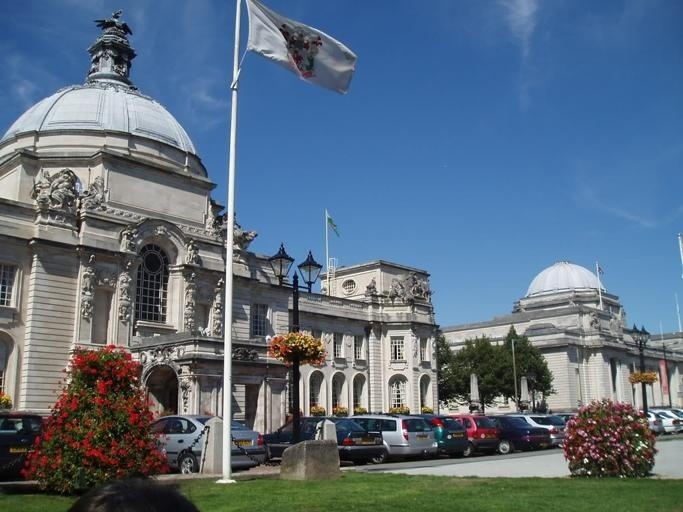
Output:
(555, 405), (681, 445)
(409, 414), (468, 458)
(0, 411), (65, 490)
(486, 416), (549, 456)
(143, 412), (269, 478)
(257, 415), (386, 467)
(442, 415), (498, 458)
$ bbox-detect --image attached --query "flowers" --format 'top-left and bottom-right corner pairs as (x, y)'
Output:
(627, 369), (660, 387)
(269, 331), (328, 371)
(305, 400), (436, 417)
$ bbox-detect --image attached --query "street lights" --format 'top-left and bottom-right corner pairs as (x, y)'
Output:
(267, 240), (324, 447)
(628, 322), (651, 425)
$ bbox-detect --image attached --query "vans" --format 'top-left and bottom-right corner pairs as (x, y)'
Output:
(509, 413), (565, 449)
(341, 412), (438, 464)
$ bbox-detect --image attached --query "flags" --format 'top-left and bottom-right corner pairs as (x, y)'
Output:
(246, 0), (358, 96)
(594, 266), (604, 276)
(327, 214), (340, 238)
